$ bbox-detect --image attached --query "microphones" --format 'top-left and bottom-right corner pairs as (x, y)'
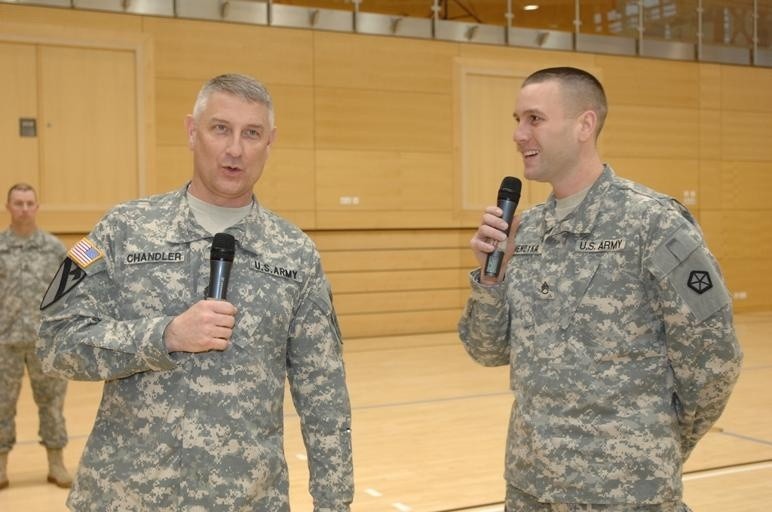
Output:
(483, 176), (522, 276)
(207, 232), (236, 300)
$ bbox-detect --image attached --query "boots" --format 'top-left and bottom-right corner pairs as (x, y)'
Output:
(0, 454), (9, 489)
(48, 449), (72, 487)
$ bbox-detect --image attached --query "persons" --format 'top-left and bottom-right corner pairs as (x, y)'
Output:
(0, 183), (76, 488)
(456, 65), (742, 512)
(35, 74), (354, 512)
